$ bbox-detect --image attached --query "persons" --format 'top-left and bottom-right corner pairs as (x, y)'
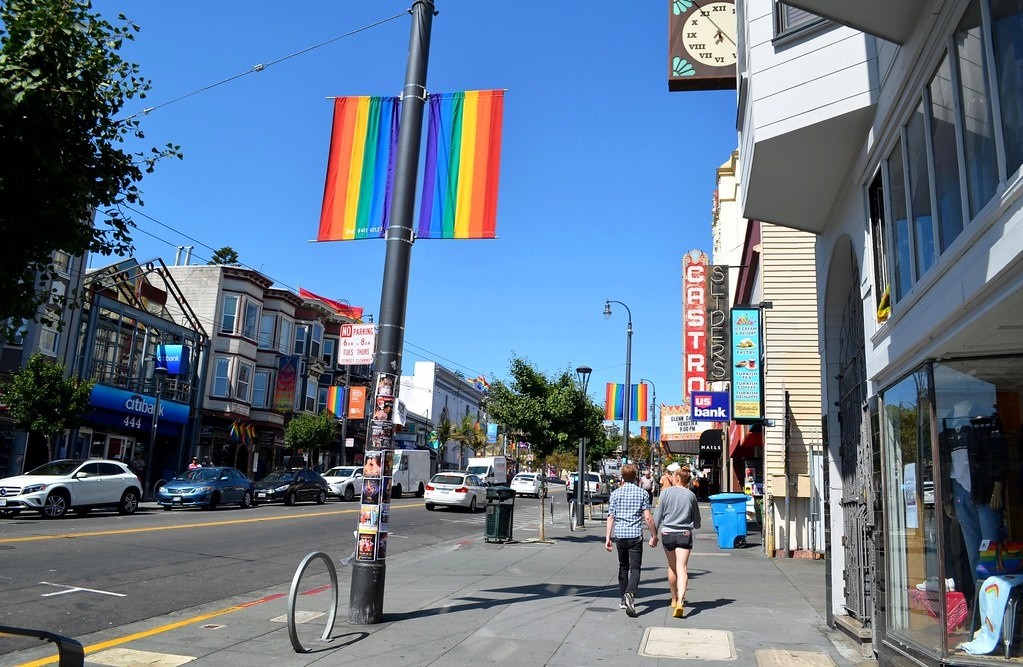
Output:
(542, 474), (548, 498)
(131, 453), (146, 483)
(611, 465), (707, 506)
(649, 469), (701, 617)
(319, 463), (326, 473)
(364, 458), (381, 476)
(187, 456), (214, 480)
(604, 465), (657, 617)
(507, 470), (513, 488)
(938, 399), (1008, 587)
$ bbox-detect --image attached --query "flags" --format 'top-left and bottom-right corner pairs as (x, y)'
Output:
(318, 97), (400, 241)
(604, 383), (625, 420)
(641, 426), (660, 447)
(468, 376), (490, 392)
(326, 386), (366, 419)
(228, 420), (256, 448)
(417, 89), (505, 239)
(630, 384), (648, 421)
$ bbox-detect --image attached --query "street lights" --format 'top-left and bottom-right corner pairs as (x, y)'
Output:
(604, 299), (634, 465)
(144, 366), (170, 502)
(575, 366), (593, 527)
(649, 403), (665, 496)
(898, 399), (919, 495)
(640, 378), (657, 470)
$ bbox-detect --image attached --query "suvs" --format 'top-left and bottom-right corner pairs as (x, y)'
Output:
(0, 459), (143, 524)
(252, 467), (327, 506)
(923, 481), (936, 504)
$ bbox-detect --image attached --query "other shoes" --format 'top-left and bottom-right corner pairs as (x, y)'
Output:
(619, 598), (627, 609)
(670, 601), (678, 608)
(673, 604), (684, 617)
(624, 593), (636, 617)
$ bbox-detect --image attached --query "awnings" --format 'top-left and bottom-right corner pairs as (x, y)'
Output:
(659, 403), (723, 457)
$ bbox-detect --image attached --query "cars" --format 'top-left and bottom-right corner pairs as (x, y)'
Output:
(509, 472), (549, 499)
(424, 469), (489, 513)
(321, 465), (363, 502)
(157, 466), (254, 512)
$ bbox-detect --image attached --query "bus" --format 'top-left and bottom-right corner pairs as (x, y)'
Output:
(599, 458), (633, 481)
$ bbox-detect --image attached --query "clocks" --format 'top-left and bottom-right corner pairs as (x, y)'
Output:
(668, 0), (737, 91)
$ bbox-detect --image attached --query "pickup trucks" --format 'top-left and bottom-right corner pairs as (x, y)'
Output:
(566, 471), (609, 503)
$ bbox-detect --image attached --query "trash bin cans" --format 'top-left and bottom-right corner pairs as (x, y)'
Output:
(708, 493), (752, 549)
(485, 487), (516, 543)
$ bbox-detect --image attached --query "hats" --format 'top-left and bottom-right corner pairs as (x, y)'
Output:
(193, 457), (198, 459)
(204, 456), (209, 459)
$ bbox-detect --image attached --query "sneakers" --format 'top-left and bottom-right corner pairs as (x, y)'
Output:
(915, 576), (956, 592)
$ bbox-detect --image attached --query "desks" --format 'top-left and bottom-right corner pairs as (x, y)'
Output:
(967, 580), (1023, 660)
(901, 587), (968, 646)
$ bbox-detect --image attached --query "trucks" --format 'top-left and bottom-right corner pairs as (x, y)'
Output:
(465, 455), (507, 487)
(391, 449), (436, 498)
